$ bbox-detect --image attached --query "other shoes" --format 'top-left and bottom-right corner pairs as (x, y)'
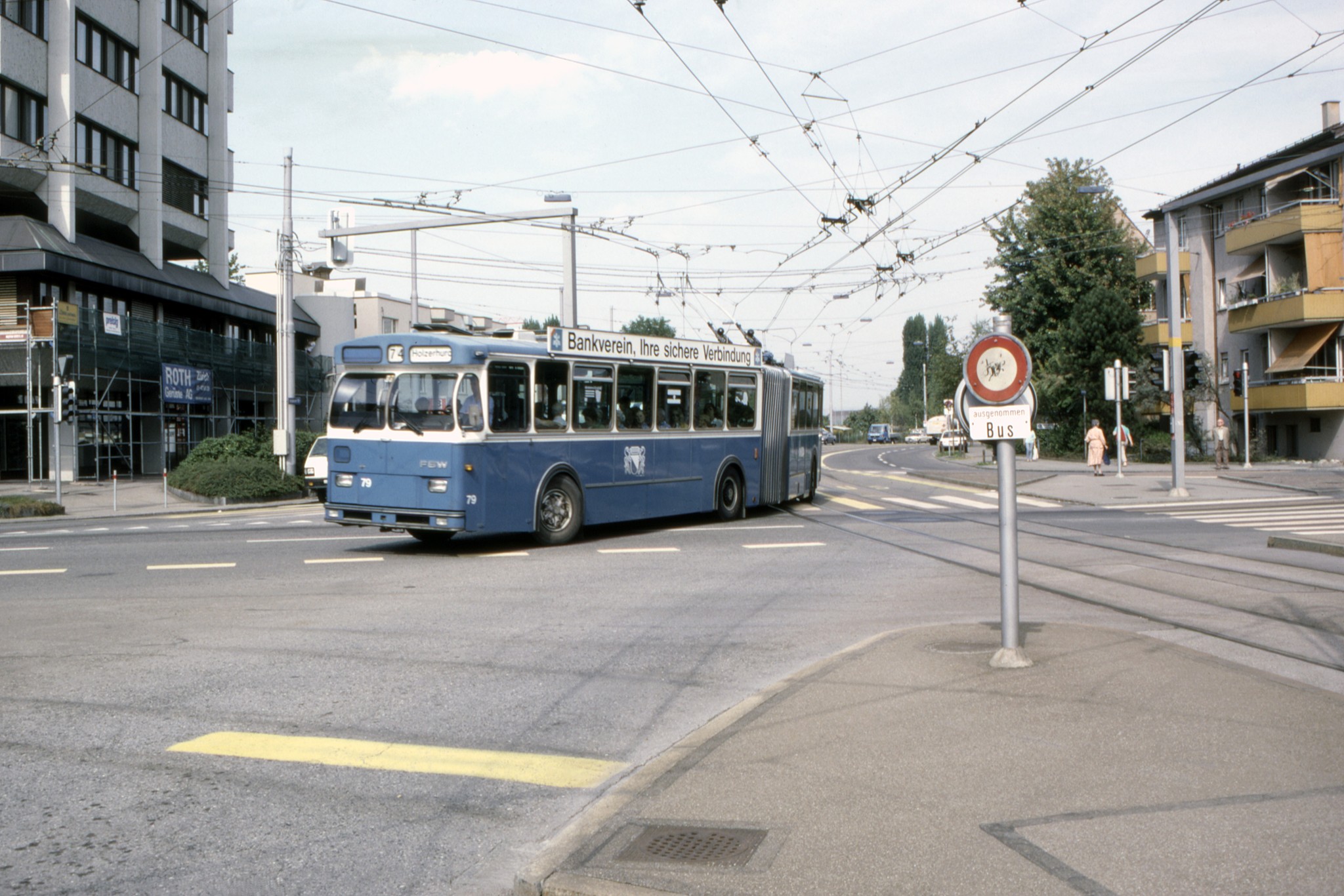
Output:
(1094, 473), (1098, 476)
(1098, 472), (1105, 476)
(1123, 462), (1127, 466)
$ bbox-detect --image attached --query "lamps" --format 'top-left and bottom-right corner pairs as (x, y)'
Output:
(832, 294), (850, 300)
(860, 318), (873, 323)
(542, 191), (572, 202)
(1076, 178), (1105, 194)
(802, 343), (812, 348)
(654, 291), (673, 298)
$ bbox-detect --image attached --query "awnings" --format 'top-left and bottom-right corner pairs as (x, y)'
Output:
(1264, 166), (1339, 193)
(1226, 256), (1266, 292)
(1264, 322), (1344, 373)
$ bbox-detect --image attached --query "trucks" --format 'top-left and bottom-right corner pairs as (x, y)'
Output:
(867, 423), (901, 444)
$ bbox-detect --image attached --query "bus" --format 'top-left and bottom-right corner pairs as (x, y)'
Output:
(322, 322), (824, 549)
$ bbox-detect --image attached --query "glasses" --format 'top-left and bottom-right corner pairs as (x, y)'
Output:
(671, 413), (681, 417)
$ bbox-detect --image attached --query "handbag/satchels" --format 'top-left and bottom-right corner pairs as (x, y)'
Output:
(1032, 445), (1039, 460)
(1102, 449), (1111, 465)
(1115, 426), (1127, 442)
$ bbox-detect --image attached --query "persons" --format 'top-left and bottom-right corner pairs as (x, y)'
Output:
(581, 407), (606, 428)
(656, 408), (671, 428)
(616, 410), (628, 429)
(459, 375), (494, 431)
(704, 404), (731, 427)
(1113, 420), (1133, 466)
(1212, 418), (1231, 469)
(1023, 430), (1036, 461)
(447, 398), (462, 414)
(415, 397), (429, 415)
(671, 407), (688, 428)
(551, 402), (566, 426)
(686, 407), (708, 427)
(534, 402), (548, 420)
(635, 410), (650, 429)
(1084, 419), (1108, 476)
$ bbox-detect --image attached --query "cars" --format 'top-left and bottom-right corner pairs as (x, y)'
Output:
(905, 431), (932, 444)
(822, 428), (837, 445)
(938, 428), (968, 454)
(303, 435), (327, 503)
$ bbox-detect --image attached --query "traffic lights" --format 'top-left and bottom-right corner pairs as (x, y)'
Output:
(1181, 349), (1199, 391)
(58, 354), (74, 378)
(1233, 369), (1242, 396)
(1121, 366), (1136, 401)
(58, 384), (74, 423)
(1150, 348), (1170, 392)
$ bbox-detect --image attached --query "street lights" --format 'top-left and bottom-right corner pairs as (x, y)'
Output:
(1081, 390), (1087, 462)
